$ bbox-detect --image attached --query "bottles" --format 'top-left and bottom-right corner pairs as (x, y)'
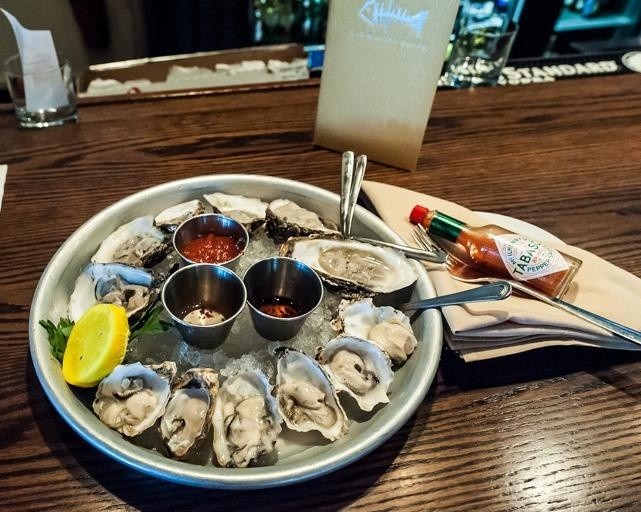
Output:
(411, 205), (584, 298)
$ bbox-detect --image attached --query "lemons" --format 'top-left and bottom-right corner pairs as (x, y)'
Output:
(63, 303), (129, 388)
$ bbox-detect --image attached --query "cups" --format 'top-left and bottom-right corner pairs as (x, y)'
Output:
(453, 9), (521, 86)
(5, 50), (76, 127)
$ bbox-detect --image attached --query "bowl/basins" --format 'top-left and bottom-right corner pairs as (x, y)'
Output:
(30, 173), (446, 488)
(162, 214), (321, 346)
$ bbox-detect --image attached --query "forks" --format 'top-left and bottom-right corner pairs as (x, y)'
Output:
(413, 226), (641, 347)
